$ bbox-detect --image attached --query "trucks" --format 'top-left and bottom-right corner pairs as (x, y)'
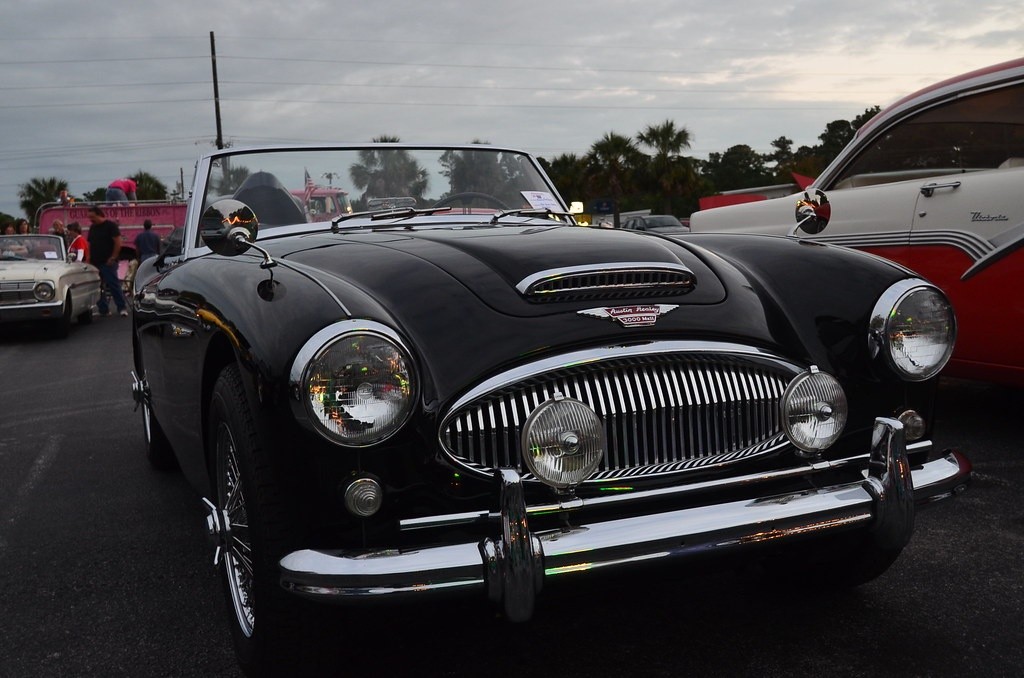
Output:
(32, 189), (353, 298)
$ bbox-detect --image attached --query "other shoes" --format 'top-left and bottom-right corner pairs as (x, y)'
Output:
(119, 309), (128, 316)
(94, 311), (111, 317)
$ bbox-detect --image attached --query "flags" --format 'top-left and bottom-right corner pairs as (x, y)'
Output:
(304, 170), (316, 204)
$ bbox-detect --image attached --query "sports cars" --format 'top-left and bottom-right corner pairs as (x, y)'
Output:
(688, 56), (1023, 393)
(127, 141), (976, 678)
(0, 233), (104, 341)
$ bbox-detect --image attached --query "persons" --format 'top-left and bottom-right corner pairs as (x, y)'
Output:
(106, 177), (140, 206)
(133, 219), (161, 265)
(51, 219), (73, 259)
(86, 206), (128, 317)
(0, 218), (37, 259)
(67, 222), (89, 264)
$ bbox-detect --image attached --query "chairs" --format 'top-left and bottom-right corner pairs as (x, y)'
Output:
(232, 172), (308, 231)
(34, 245), (56, 260)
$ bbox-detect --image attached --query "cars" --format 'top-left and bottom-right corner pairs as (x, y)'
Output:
(622, 214), (690, 234)
